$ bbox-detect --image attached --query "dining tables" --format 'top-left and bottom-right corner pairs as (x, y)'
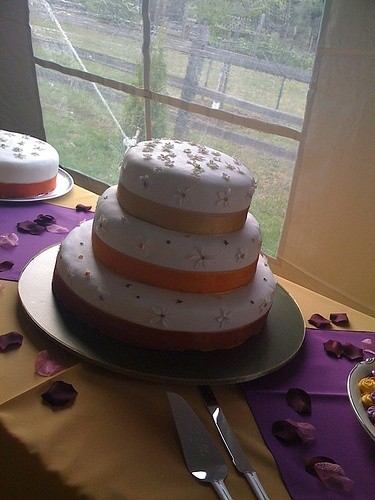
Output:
(0, 185), (375, 500)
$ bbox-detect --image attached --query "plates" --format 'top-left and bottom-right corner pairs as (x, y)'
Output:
(347, 358), (375, 442)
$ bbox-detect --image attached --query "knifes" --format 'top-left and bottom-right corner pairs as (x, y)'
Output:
(199, 384), (271, 500)
